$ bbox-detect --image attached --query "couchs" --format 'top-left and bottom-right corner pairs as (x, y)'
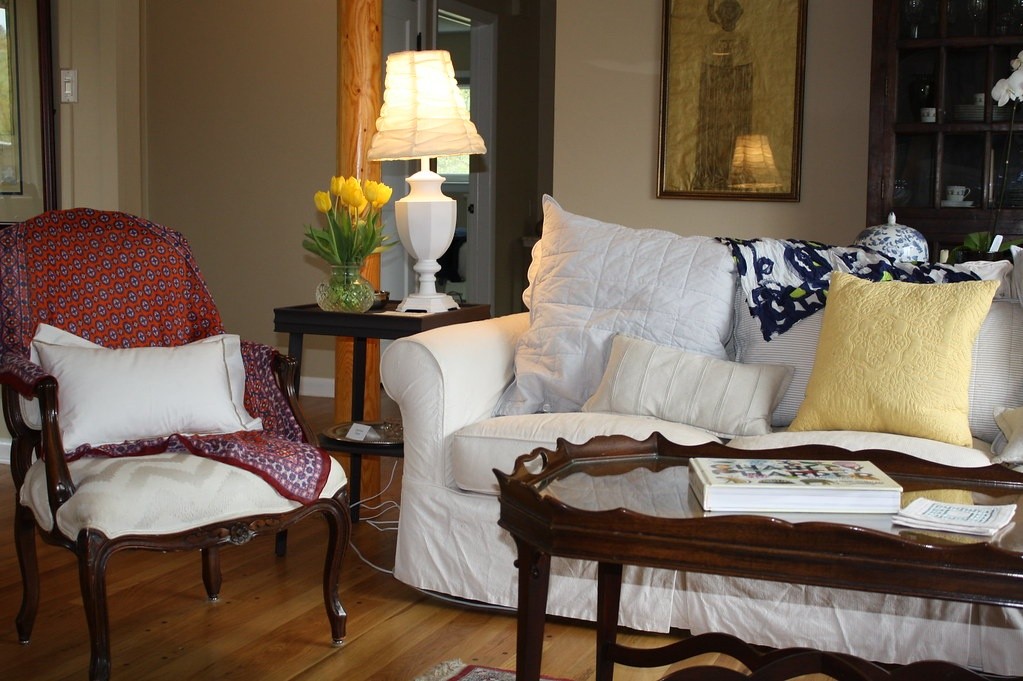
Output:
(378, 311), (1023, 677)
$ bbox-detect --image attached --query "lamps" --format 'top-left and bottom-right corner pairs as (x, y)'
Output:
(369, 49), (489, 314)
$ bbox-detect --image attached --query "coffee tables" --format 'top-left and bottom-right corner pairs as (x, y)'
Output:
(491, 431), (1023, 681)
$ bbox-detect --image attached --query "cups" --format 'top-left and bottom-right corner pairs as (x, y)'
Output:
(974, 93), (985, 103)
(920, 107), (936, 122)
(946, 186), (971, 201)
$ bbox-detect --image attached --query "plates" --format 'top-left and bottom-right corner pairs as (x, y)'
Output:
(321, 421), (403, 445)
(941, 200), (974, 207)
(951, 104), (1011, 123)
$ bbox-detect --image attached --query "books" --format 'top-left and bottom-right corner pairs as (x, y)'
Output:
(688, 457), (904, 515)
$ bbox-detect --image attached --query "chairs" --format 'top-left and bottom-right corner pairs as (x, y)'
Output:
(0, 207), (351, 681)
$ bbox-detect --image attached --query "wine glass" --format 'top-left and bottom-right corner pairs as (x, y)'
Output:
(904, 0), (1023, 37)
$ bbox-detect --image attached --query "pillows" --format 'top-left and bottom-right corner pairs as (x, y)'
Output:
(727, 277), (826, 426)
(960, 298), (1023, 443)
(30, 334), (263, 460)
(580, 332), (795, 436)
(494, 193), (737, 417)
(790, 270), (1001, 448)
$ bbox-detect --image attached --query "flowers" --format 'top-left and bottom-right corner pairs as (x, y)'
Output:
(302, 174), (401, 310)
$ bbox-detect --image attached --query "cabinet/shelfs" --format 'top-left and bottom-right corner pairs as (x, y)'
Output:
(864, 0), (1023, 263)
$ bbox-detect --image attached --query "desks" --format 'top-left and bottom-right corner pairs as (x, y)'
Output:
(268, 298), (490, 558)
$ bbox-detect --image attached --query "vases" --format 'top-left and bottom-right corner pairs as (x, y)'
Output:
(317, 269), (374, 313)
(907, 73), (935, 123)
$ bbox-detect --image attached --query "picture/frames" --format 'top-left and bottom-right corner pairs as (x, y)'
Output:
(657, 0), (808, 201)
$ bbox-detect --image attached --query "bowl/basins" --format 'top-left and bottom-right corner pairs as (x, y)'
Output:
(373, 288), (390, 311)
